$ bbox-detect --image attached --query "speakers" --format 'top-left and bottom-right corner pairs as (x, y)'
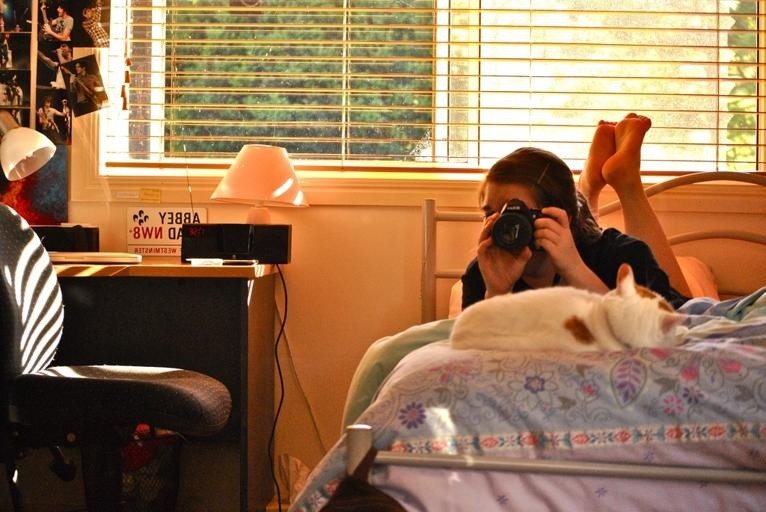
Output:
(181, 224), (291, 264)
(30, 225), (99, 252)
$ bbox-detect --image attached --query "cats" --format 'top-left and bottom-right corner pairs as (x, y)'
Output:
(448, 263), (690, 354)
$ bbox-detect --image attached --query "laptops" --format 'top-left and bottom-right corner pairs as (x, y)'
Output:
(49, 252), (142, 264)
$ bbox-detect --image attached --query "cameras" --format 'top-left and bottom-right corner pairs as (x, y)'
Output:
(486, 199), (552, 251)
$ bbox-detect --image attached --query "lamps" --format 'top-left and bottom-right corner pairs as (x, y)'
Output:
(208, 144), (312, 225)
(0, 108), (56, 184)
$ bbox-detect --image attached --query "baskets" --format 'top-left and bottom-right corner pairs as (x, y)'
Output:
(81, 437), (180, 512)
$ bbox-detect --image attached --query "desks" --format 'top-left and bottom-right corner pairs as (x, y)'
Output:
(49, 251), (290, 511)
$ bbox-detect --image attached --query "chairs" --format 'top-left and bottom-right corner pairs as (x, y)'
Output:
(0, 204), (237, 511)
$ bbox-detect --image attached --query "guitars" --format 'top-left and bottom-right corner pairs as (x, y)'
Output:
(39, 0), (57, 42)
(60, 66), (103, 108)
(35, 109), (59, 134)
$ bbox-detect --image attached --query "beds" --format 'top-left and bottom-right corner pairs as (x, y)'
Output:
(324, 168), (766, 511)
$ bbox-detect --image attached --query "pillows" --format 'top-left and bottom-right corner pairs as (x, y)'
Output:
(448, 237), (721, 318)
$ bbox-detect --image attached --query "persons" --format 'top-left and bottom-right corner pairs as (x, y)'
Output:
(0, 0), (117, 145)
(462, 113), (694, 314)
